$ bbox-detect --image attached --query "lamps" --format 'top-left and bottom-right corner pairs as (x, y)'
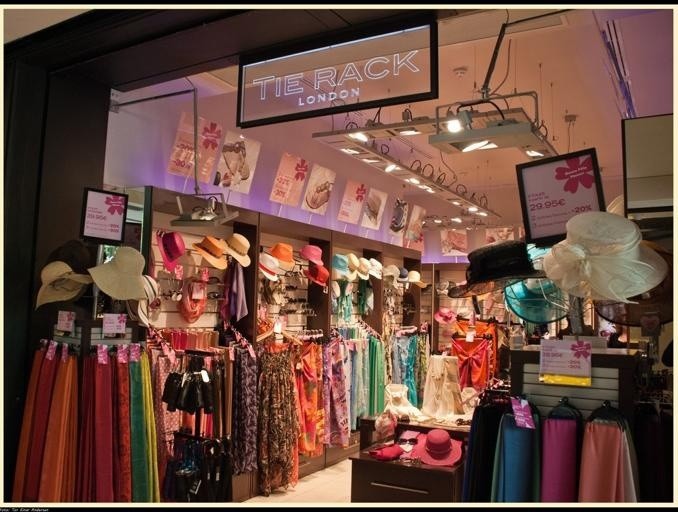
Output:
(311, 37), (576, 230)
(111, 88), (240, 228)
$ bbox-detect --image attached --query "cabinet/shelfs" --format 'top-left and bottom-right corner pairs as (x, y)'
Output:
(421, 263), (524, 502)
(144, 185), (421, 503)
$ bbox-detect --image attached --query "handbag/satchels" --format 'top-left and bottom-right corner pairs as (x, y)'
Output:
(368, 444), (404, 461)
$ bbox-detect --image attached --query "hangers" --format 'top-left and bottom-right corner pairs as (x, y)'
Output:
(484, 389), (627, 432)
(254, 317), (304, 346)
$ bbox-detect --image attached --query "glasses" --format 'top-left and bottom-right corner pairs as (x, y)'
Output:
(395, 437), (418, 445)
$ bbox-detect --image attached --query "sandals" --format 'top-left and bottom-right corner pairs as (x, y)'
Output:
(222, 140), (250, 181)
(306, 181), (335, 210)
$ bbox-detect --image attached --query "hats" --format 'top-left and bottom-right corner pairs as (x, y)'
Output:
(434, 308), (456, 325)
(258, 242), (421, 287)
(87, 247), (147, 302)
(364, 191), (382, 226)
(447, 210), (672, 323)
(34, 260), (93, 311)
(192, 235), (228, 270)
(126, 274), (159, 328)
(415, 429), (462, 465)
(156, 229), (185, 271)
(223, 233), (251, 268)
